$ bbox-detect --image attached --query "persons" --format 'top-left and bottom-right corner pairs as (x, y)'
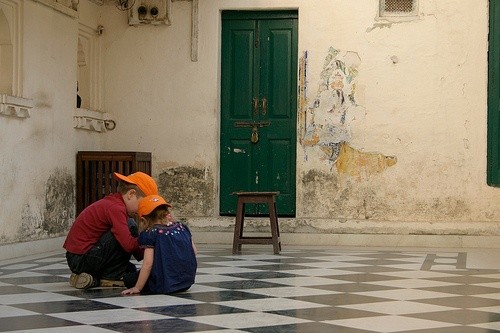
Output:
(63, 170), (159, 289)
(121, 194), (197, 296)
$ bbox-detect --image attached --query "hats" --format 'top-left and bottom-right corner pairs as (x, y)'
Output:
(114, 172), (159, 196)
(138, 194), (172, 218)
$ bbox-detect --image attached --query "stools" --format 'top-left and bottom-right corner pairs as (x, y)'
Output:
(231, 191), (282, 254)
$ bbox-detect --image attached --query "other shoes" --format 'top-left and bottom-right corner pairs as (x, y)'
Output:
(68, 272), (97, 289)
(100, 276), (125, 287)
